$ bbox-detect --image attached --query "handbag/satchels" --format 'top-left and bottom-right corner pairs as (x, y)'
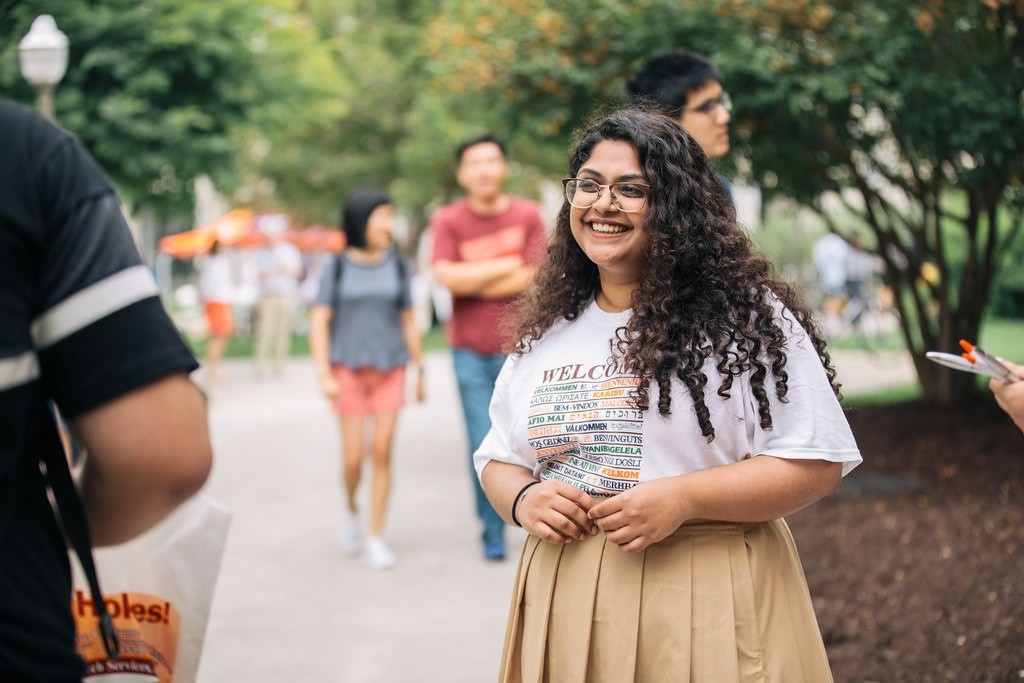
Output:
(46, 458), (234, 683)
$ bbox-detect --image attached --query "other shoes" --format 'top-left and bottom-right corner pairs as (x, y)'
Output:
(480, 528), (506, 560)
(361, 530), (397, 573)
(339, 509), (365, 556)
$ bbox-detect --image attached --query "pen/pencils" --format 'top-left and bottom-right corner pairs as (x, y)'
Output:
(960, 337), (1022, 383)
(924, 350), (1003, 380)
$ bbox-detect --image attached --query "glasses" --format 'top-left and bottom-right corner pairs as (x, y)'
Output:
(561, 177), (653, 213)
(683, 89), (733, 120)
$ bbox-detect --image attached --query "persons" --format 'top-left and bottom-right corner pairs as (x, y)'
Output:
(432, 135), (551, 564)
(0, 102), (212, 683)
(988, 356), (1024, 432)
(196, 215), (304, 382)
(474, 111), (844, 683)
(815, 228), (886, 336)
(625, 51), (739, 199)
(307, 189), (428, 571)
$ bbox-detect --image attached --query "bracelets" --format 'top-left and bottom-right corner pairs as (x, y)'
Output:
(415, 363), (429, 377)
(511, 477), (544, 532)
